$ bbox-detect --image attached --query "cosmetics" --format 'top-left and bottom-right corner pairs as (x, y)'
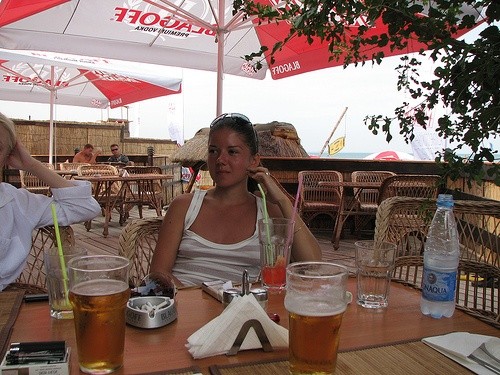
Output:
(222, 289), (268, 308)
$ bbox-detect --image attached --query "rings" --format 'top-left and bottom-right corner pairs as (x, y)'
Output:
(265, 172), (270, 175)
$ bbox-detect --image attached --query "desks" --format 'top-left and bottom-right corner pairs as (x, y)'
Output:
(317, 180), (428, 252)
(73, 172), (174, 238)
(54, 171), (78, 180)
(0, 270), (500, 375)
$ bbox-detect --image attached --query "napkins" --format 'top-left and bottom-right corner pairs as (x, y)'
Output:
(421, 331), (500, 375)
(122, 170), (130, 177)
(186, 290), (293, 359)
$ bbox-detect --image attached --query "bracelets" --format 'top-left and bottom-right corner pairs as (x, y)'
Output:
(294, 222), (305, 232)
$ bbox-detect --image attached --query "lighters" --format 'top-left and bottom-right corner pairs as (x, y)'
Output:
(6, 352), (63, 362)
(10, 340), (65, 354)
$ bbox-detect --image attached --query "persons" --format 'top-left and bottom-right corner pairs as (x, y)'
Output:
(106, 144), (129, 166)
(0, 112), (101, 293)
(73, 143), (102, 163)
(149, 112), (322, 288)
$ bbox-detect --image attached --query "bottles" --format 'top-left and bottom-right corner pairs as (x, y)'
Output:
(221, 289), (238, 312)
(420, 194), (459, 319)
(251, 288), (269, 313)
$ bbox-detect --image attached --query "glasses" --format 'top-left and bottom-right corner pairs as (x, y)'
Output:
(112, 149), (118, 151)
(210, 112), (258, 151)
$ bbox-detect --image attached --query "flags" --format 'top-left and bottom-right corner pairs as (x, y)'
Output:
(400, 90), (445, 161)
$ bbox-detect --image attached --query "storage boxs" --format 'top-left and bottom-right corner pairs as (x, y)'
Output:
(0, 347), (71, 375)
(202, 278), (232, 302)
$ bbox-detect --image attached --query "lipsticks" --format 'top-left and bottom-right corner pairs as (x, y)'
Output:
(242, 268), (249, 294)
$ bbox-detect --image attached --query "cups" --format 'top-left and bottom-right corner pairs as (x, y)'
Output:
(283, 261), (348, 375)
(258, 218), (295, 293)
(42, 246), (87, 319)
(354, 240), (397, 309)
(67, 255), (131, 375)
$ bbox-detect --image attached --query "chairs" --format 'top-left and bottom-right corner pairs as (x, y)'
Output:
(6, 161), (500, 330)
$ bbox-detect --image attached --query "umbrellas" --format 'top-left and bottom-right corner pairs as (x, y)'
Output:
(0, 48), (182, 169)
(0, 0), (500, 118)
(365, 151), (414, 160)
